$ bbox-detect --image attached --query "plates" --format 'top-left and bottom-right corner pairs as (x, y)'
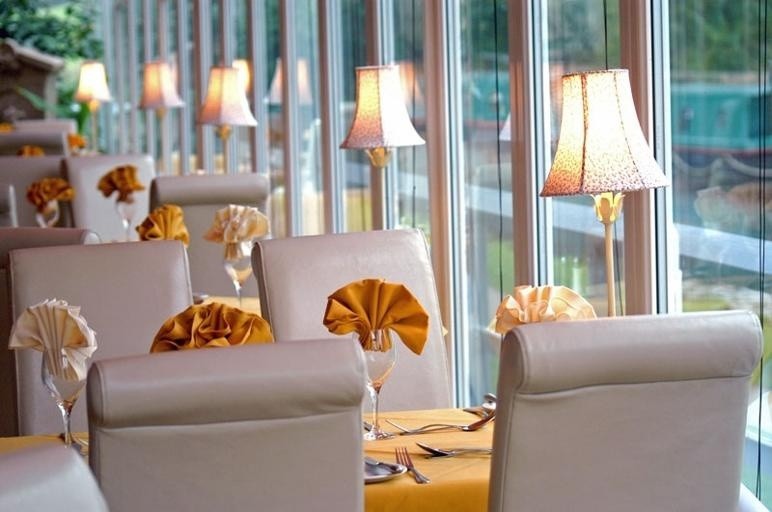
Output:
(363, 461), (406, 486)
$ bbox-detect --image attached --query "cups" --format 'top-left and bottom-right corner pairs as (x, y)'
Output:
(559, 254), (590, 296)
(34, 199), (61, 227)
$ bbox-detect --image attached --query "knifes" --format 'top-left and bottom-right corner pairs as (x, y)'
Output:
(363, 455), (402, 474)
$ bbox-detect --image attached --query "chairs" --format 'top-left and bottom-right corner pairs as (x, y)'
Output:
(14, 119), (78, 135)
(0, 155), (74, 233)
(63, 155), (156, 242)
(488, 309), (764, 512)
(0, 125), (67, 155)
(10, 237), (195, 436)
(251, 229), (453, 414)
(86, 337), (368, 512)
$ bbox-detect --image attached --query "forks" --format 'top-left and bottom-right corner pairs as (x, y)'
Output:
(396, 446), (430, 483)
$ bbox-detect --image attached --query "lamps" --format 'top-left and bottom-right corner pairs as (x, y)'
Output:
(196, 64), (258, 170)
(138, 60), (187, 168)
(338, 63), (430, 224)
(76, 60), (114, 150)
(538, 67), (664, 313)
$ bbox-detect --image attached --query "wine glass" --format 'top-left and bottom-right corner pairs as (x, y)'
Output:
(223, 241), (254, 312)
(40, 348), (90, 459)
(116, 193), (138, 242)
(353, 328), (400, 440)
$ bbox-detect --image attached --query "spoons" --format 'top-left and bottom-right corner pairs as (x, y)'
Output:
(385, 410), (496, 434)
(362, 420), (458, 439)
(415, 442), (494, 457)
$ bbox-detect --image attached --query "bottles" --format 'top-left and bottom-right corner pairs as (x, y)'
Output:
(1, 180), (20, 228)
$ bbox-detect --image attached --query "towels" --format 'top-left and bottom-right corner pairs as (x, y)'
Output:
(18, 143), (45, 154)
(26, 176), (73, 211)
(7, 299), (98, 382)
(323, 280), (429, 355)
(492, 282), (597, 334)
(69, 133), (85, 149)
(149, 301), (274, 355)
